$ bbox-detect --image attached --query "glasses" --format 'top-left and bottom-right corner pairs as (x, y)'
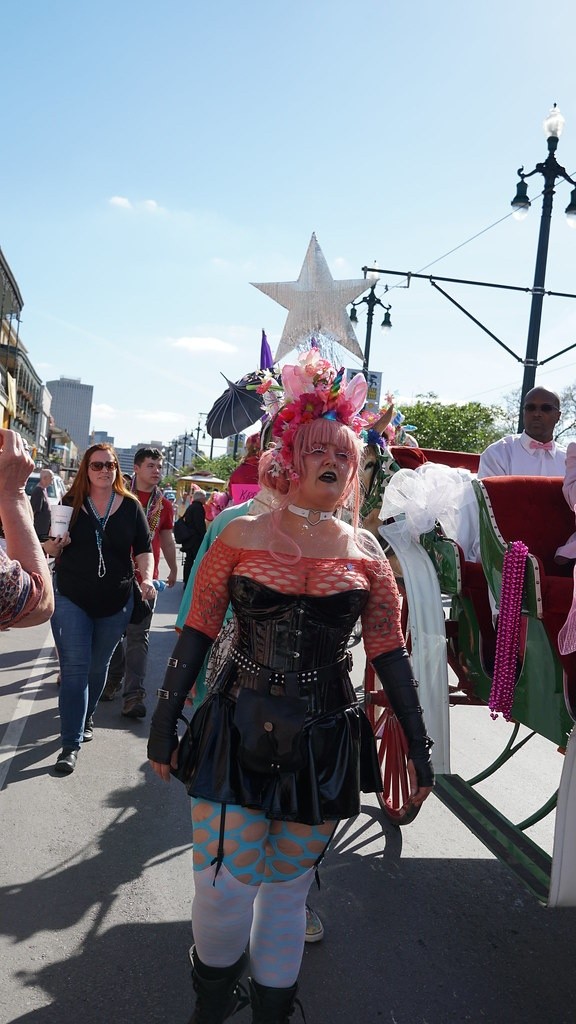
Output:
(90, 462), (118, 472)
(523, 403), (559, 413)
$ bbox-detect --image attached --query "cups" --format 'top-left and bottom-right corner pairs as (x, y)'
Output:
(50, 505), (74, 539)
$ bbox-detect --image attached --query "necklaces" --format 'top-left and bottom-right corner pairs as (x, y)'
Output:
(487, 540), (528, 721)
(86, 489), (116, 578)
(287, 503), (334, 526)
(126, 474), (163, 563)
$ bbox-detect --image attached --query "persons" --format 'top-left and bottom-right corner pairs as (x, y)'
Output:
(144, 422), (436, 1024)
(554, 440), (576, 681)
(42, 443), (158, 772)
(172, 432), (450, 714)
(478, 387), (567, 628)
(0, 427), (56, 631)
(29, 469), (54, 559)
(120, 471), (132, 490)
(99, 447), (178, 717)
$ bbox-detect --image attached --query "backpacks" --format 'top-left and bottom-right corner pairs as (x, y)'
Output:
(173, 514), (196, 552)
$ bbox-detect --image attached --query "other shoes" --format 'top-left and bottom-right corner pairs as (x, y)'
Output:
(304, 905), (325, 943)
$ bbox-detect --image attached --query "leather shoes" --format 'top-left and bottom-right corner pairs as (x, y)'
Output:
(83, 715), (94, 742)
(56, 748), (78, 774)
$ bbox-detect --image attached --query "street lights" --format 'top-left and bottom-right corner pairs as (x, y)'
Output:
(509, 99), (575, 433)
(350, 258), (394, 413)
(161, 430), (192, 476)
(189, 417), (206, 462)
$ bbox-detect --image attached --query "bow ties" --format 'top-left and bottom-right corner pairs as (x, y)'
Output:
(529, 441), (553, 451)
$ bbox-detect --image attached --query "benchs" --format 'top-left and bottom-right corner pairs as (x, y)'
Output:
(385, 436), (576, 680)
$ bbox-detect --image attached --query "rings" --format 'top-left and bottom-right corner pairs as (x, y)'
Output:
(24, 443), (29, 451)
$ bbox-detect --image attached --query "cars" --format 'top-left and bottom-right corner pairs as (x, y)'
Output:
(22, 472), (67, 507)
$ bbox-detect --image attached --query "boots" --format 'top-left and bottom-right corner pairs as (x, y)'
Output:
(247, 976), (297, 1024)
(188, 945), (246, 1024)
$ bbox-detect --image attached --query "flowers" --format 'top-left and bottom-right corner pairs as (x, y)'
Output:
(243, 347), (380, 480)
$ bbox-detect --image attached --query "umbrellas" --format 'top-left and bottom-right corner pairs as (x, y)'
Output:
(259, 326), (273, 370)
(206, 368), (277, 439)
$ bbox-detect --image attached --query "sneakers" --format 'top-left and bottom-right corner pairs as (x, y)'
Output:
(122, 690), (147, 719)
(100, 681), (121, 702)
